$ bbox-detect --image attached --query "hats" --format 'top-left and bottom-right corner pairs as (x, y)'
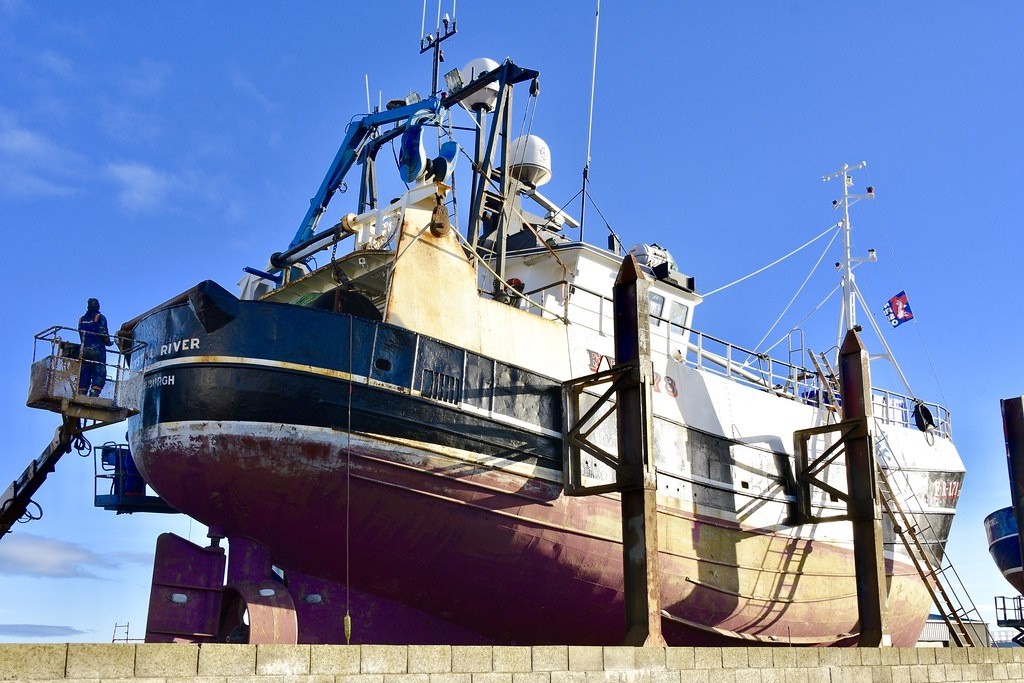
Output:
(87, 298), (100, 310)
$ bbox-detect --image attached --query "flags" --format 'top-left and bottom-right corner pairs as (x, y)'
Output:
(882, 290), (913, 328)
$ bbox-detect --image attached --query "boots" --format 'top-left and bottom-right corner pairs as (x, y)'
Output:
(89, 390), (99, 398)
(77, 390), (88, 395)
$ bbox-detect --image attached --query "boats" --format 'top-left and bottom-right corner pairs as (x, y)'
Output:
(92, 1), (968, 648)
(982, 504), (1024, 594)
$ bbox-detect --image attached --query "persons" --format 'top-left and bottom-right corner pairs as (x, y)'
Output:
(78, 298), (114, 397)
